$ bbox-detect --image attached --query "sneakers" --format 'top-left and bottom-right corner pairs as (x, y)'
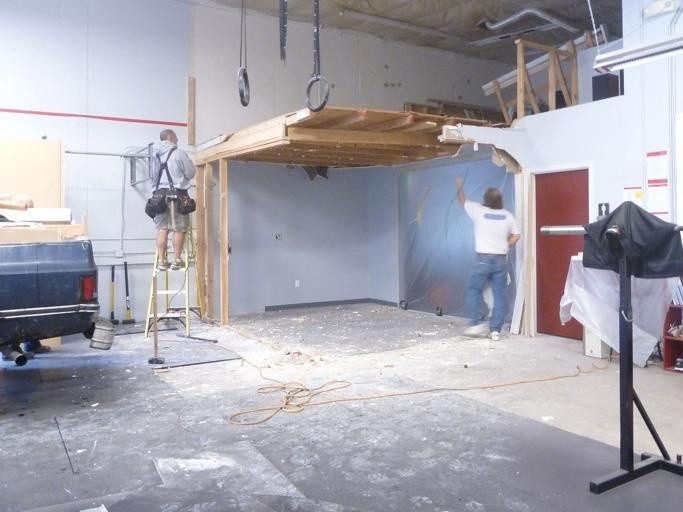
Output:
(23, 345), (51, 354)
(459, 323), (488, 338)
(156, 260), (170, 271)
(488, 328), (501, 341)
(2, 350), (35, 361)
(674, 358), (683, 374)
(171, 261), (185, 271)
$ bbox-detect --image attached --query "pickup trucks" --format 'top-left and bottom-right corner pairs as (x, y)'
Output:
(0, 241), (115, 365)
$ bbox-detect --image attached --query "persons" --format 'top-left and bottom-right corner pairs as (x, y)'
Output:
(149, 128), (197, 270)
(2, 339), (51, 361)
(456, 175), (521, 341)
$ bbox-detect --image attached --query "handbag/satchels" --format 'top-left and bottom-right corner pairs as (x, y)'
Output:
(176, 193), (197, 215)
(145, 189), (170, 217)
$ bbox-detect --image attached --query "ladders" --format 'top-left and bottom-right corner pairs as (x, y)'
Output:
(145, 222), (204, 338)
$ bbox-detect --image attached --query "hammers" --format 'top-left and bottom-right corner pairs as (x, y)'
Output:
(110, 262), (135, 324)
